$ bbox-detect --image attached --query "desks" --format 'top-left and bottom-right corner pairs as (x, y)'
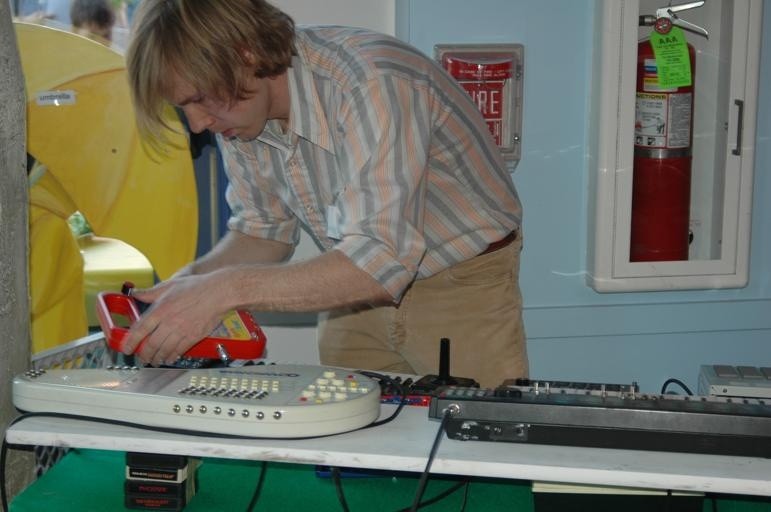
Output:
(6, 372), (771, 498)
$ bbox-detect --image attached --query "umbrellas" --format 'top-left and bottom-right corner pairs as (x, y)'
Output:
(12, 20), (198, 281)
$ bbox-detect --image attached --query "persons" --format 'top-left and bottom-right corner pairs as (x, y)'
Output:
(24, 0), (115, 46)
(120, 2), (531, 390)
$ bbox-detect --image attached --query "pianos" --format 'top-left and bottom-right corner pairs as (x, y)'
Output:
(428, 383), (771, 459)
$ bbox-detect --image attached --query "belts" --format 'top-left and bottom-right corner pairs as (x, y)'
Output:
(476, 229), (518, 257)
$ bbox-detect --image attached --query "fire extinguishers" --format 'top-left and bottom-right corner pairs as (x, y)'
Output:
(630, 0), (709, 262)
